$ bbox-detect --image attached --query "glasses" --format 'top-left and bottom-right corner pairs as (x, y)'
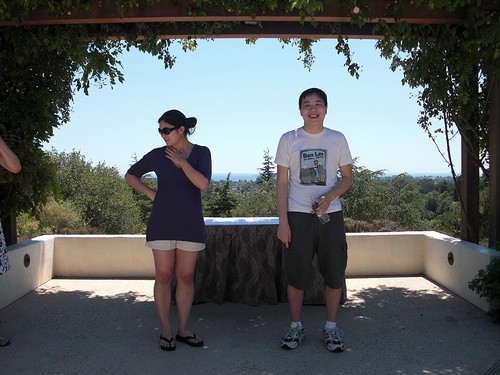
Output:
(158, 125), (179, 135)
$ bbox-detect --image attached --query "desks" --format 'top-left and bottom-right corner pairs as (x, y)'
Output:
(170, 217), (348, 305)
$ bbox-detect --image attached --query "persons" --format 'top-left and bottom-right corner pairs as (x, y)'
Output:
(125, 109), (211, 351)
(0, 136), (21, 346)
(276, 88), (353, 353)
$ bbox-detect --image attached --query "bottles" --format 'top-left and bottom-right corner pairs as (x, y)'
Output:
(312, 201), (330, 224)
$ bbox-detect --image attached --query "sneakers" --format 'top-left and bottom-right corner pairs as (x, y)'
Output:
(323, 327), (346, 352)
(281, 322), (305, 350)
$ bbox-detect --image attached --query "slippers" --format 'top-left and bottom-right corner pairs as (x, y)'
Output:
(175, 332), (204, 347)
(160, 335), (177, 351)
